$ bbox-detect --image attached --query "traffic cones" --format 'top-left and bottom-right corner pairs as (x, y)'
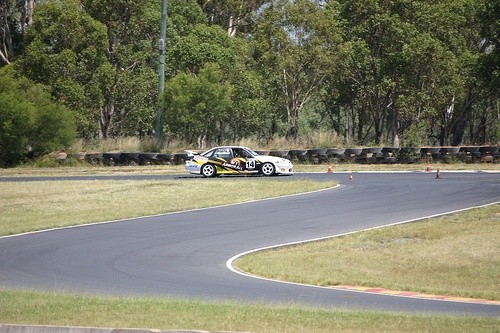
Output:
(434, 168), (443, 179)
(427, 162), (431, 171)
(327, 163), (332, 172)
(349, 172), (353, 181)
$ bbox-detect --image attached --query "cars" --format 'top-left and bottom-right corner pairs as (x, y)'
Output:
(184, 146), (294, 178)
